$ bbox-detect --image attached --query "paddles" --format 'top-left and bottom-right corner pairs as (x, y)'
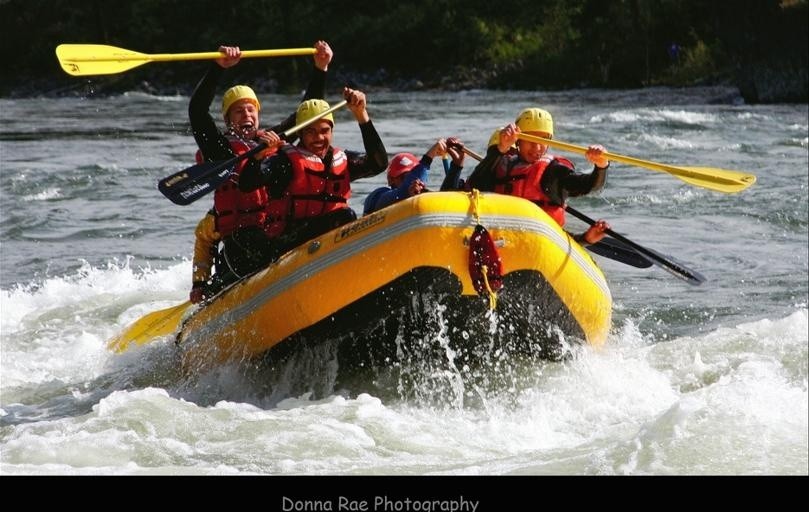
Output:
(450, 139), (707, 288)
(55, 43), (319, 76)
(158, 98), (351, 208)
(561, 229), (655, 269)
(514, 131), (757, 194)
(101, 298), (190, 353)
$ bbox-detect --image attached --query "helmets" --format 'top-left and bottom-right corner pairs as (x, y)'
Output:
(295, 98), (335, 130)
(516, 106), (554, 140)
(221, 85), (262, 118)
(386, 151), (422, 179)
(487, 125), (518, 149)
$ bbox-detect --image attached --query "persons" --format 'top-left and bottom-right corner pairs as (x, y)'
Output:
(465, 107), (610, 228)
(408, 178), (425, 196)
(238, 85), (389, 253)
(488, 128), (517, 158)
(363, 138), (447, 217)
(440, 137), (610, 247)
(188, 40), (333, 273)
(190, 204), (222, 305)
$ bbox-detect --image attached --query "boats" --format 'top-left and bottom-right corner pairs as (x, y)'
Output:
(106, 190), (611, 379)
(153, 191), (611, 413)
